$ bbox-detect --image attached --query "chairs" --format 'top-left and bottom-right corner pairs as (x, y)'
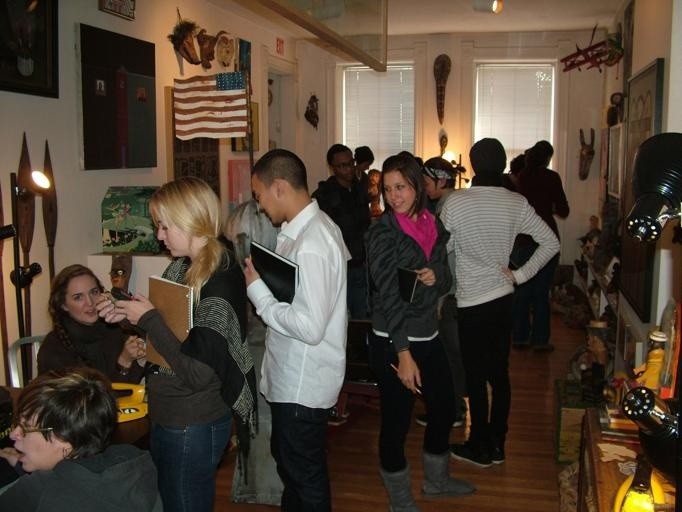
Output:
(336, 317), (380, 421)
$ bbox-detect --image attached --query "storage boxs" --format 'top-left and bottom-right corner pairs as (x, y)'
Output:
(553, 378), (601, 464)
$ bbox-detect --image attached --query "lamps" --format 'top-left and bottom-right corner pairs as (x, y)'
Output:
(491, 0), (505, 14)
(0, 170), (50, 386)
(620, 131), (682, 512)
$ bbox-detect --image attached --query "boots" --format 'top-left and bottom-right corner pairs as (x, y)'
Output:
(379, 464), (421, 512)
(421, 451), (476, 497)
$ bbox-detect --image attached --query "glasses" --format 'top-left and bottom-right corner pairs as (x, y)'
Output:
(11, 422), (54, 437)
(331, 161), (355, 169)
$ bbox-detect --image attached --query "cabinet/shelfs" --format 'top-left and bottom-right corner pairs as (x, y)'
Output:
(84, 252), (177, 301)
(575, 407), (682, 512)
(614, 292), (648, 385)
(571, 242), (619, 348)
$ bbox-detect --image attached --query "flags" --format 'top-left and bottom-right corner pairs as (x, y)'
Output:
(172, 69), (250, 142)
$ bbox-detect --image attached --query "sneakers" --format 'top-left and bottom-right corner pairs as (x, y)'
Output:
(448, 443), (493, 468)
(533, 344), (554, 352)
(491, 443), (505, 465)
(415, 414), (466, 427)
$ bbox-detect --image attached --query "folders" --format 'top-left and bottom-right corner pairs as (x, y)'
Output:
(249, 240), (300, 304)
(395, 264), (420, 304)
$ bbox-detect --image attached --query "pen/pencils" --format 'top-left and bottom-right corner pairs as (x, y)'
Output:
(389, 361), (422, 395)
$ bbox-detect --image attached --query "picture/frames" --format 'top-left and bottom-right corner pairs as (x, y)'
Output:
(79, 22), (158, 170)
(0, 1), (59, 99)
(609, 124), (622, 197)
(228, 159), (257, 209)
(617, 57), (664, 324)
(232, 102), (259, 153)
(98, 0), (137, 23)
(163, 86), (221, 203)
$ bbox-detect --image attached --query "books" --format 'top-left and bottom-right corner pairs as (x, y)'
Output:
(247, 239), (300, 327)
(596, 401), (642, 446)
(368, 261), (419, 305)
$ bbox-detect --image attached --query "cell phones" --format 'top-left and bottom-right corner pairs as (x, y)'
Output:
(109, 286), (135, 300)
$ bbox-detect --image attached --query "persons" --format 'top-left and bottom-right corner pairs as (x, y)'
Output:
(0, 366), (164, 512)
(412, 158), (469, 431)
(311, 144), (371, 233)
(509, 141), (570, 353)
(94, 175), (248, 512)
(632, 330), (667, 397)
(441, 139), (561, 470)
(354, 146), (375, 193)
(35, 262), (145, 384)
(365, 152), (475, 511)
(242, 148), (355, 512)
